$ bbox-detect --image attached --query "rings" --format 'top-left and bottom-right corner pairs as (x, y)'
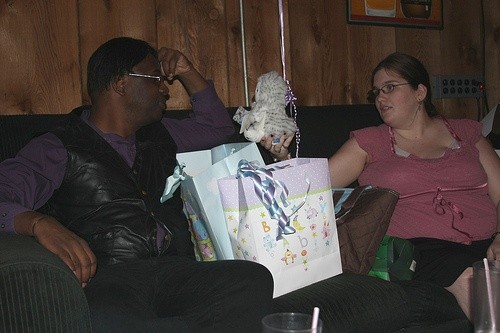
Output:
(271, 137), (280, 146)
(282, 144), (288, 149)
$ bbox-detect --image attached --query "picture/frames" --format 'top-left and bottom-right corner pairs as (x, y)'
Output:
(347, 0), (444, 31)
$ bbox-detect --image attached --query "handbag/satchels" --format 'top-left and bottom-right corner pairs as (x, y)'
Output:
(159, 142), (266, 262)
(217, 157), (343, 299)
(332, 184), (400, 277)
(368, 234), (417, 282)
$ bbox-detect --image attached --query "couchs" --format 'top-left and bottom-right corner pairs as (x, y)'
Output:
(0, 103), (385, 333)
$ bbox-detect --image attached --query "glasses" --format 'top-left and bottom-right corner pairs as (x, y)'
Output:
(120, 71), (164, 86)
(371, 81), (413, 101)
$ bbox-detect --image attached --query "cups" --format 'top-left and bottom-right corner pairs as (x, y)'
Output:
(472, 260), (500, 333)
(261, 312), (324, 333)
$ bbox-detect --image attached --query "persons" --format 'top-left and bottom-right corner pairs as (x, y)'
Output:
(0, 37), (274, 333)
(259, 52), (500, 326)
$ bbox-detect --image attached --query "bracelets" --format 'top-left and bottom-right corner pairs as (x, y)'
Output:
(32, 217), (49, 234)
(270, 153), (290, 161)
(491, 232), (500, 241)
